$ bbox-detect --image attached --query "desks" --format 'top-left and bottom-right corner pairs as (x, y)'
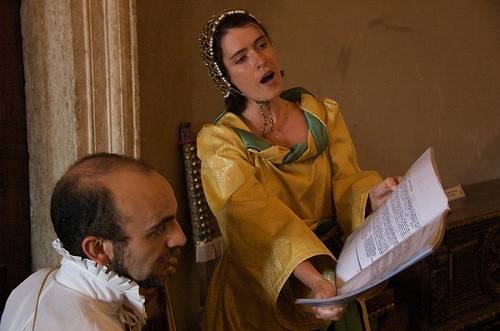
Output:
(383, 175), (500, 331)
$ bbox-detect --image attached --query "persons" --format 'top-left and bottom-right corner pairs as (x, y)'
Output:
(0, 152), (186, 331)
(196, 8), (402, 331)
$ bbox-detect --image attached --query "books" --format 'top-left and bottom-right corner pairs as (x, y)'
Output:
(295, 147), (451, 308)
